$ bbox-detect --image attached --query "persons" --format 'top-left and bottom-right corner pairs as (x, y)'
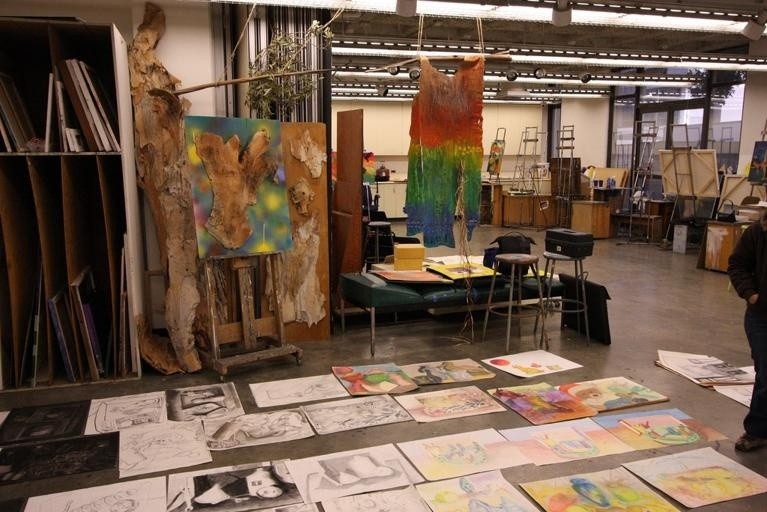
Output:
(728, 210), (767, 450)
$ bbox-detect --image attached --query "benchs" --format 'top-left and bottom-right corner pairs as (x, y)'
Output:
(339, 271), (566, 358)
(612, 213), (662, 241)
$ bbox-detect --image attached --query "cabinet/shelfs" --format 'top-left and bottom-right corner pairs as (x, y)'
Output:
(0, 16), (147, 410)
(502, 193), (610, 240)
(644, 199), (674, 239)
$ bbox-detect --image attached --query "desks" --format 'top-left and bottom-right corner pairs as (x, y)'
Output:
(702, 220), (755, 274)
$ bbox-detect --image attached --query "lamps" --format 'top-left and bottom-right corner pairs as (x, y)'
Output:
(395, 0), (416, 18)
(551, 0), (571, 28)
(374, 62), (591, 98)
(739, 9), (767, 41)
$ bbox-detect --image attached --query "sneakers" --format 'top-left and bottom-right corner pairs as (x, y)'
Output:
(733, 431), (765, 452)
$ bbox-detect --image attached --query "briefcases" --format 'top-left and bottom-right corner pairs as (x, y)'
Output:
(544, 227), (596, 260)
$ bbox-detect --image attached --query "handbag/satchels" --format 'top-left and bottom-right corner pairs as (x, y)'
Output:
(715, 197), (737, 223)
(558, 271), (614, 345)
(490, 231), (538, 276)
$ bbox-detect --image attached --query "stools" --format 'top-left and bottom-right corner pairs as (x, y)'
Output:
(361, 222), (393, 268)
(479, 250), (589, 354)
